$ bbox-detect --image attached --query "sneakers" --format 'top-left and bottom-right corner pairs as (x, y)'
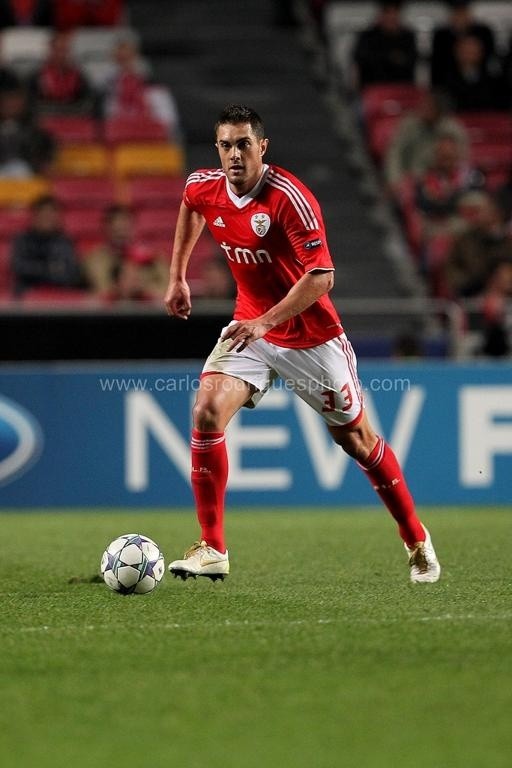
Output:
(168, 540), (229, 581)
(404, 521), (441, 583)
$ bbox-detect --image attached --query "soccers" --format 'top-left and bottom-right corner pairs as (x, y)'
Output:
(101, 535), (167, 596)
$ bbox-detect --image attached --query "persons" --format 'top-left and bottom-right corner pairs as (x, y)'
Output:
(13, 196), (92, 297)
(1, 0), (180, 185)
(353, 1), (512, 358)
(164, 101), (441, 587)
(82, 204), (170, 300)
